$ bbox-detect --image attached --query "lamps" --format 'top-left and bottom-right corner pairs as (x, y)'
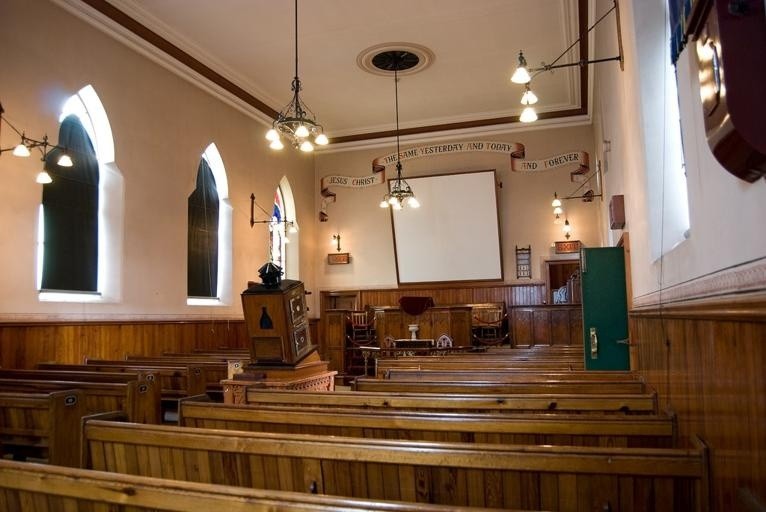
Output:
(0, 99), (79, 185)
(551, 159), (606, 216)
(265, 0), (421, 214)
(508, 1), (624, 125)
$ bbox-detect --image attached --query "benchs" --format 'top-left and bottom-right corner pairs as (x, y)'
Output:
(0, 337), (712, 510)
